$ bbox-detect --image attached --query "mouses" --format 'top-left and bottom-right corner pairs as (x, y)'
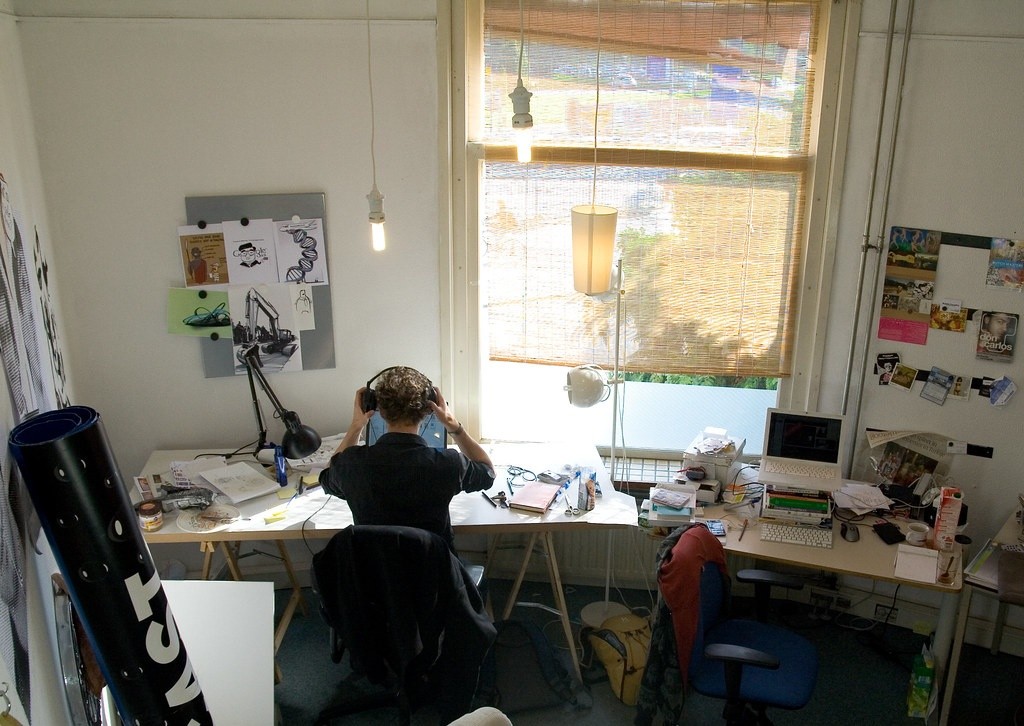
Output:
(840, 522), (860, 542)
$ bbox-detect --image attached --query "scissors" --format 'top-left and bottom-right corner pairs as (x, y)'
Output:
(563, 494), (581, 515)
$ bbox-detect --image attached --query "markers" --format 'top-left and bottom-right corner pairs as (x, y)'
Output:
(725, 498), (760, 511)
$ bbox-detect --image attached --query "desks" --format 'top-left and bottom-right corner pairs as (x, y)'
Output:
(129, 442), (640, 686)
(939, 502), (1024, 726)
(649, 494), (962, 726)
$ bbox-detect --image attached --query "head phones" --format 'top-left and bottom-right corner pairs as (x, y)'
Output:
(360, 366), (438, 415)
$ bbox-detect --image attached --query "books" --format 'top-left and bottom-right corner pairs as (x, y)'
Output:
(638, 430), (749, 546)
(199, 462), (281, 506)
(830, 483), (1024, 596)
(509, 481), (561, 514)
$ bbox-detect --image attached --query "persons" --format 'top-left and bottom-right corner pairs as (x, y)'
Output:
(319, 365), (498, 607)
(953, 377), (963, 397)
(978, 312), (1011, 354)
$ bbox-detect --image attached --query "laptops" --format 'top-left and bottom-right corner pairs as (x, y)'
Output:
(758, 408), (846, 493)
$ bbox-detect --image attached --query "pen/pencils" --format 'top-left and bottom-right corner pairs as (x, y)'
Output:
(506, 477), (515, 495)
(738, 518), (748, 542)
(481, 491), (497, 507)
(298, 475), (304, 495)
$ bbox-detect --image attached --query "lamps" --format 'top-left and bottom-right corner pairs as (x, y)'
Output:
(365, 0), (387, 250)
(508, 0), (534, 166)
(569, 0), (618, 296)
(561, 260), (634, 632)
(193, 354), (322, 467)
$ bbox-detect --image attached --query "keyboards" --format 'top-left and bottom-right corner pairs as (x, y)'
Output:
(759, 523), (832, 549)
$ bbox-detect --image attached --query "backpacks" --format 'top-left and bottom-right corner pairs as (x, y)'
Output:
(584, 613), (652, 706)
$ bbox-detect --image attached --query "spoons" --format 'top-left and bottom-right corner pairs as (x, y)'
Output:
(941, 557), (954, 577)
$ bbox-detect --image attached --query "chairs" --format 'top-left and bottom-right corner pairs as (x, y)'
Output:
(309, 524), (497, 726)
(687, 561), (820, 726)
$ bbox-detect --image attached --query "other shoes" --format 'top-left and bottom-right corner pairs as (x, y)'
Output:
(367, 675), (393, 690)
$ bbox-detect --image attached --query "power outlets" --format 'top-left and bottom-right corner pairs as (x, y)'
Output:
(873, 603), (899, 624)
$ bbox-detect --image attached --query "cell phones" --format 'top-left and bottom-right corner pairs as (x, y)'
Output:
(706, 520), (726, 536)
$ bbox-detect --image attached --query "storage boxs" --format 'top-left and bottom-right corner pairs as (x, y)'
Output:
(696, 479), (721, 503)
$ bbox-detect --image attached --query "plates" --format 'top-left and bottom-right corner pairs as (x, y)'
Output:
(176, 505), (241, 534)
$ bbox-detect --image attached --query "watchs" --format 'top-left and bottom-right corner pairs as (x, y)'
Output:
(448, 422), (463, 437)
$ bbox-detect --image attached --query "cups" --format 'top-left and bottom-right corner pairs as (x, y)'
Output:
(906, 523), (930, 547)
(936, 547), (961, 586)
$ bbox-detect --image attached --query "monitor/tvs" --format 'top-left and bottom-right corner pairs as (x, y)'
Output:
(367, 402), (450, 449)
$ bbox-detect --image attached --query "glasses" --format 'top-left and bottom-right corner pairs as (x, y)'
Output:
(508, 465), (537, 484)
